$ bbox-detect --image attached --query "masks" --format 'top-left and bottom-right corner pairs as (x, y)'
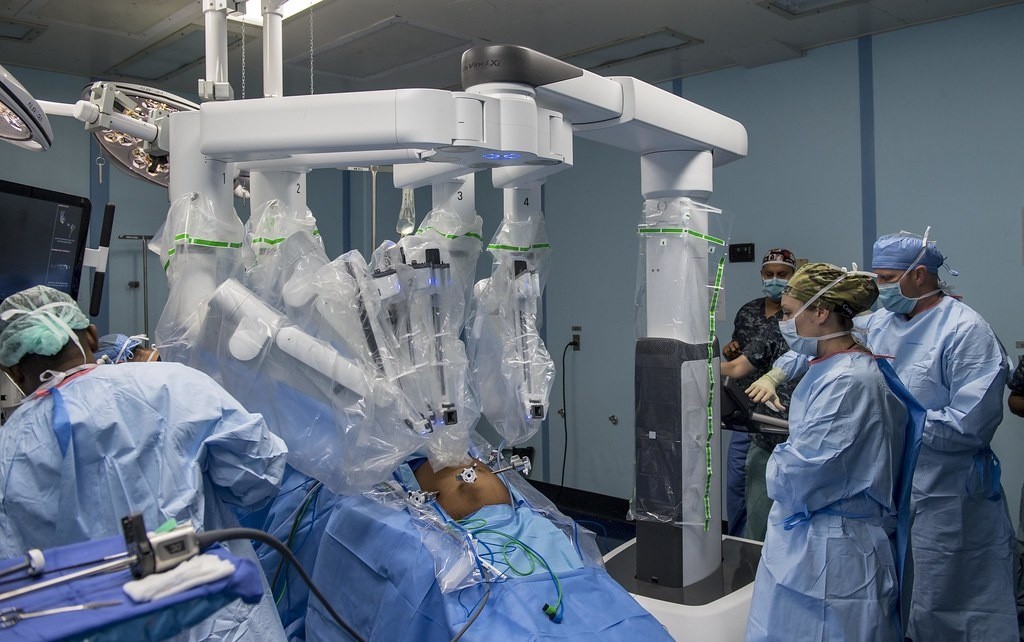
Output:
(762, 277), (788, 300)
(778, 318), (818, 356)
(877, 282), (918, 313)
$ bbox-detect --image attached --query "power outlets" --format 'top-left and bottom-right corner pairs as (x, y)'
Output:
(573, 334), (580, 351)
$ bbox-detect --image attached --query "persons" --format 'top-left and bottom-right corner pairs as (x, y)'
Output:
(720, 235), (1024, 642)
(1, 285), (514, 642)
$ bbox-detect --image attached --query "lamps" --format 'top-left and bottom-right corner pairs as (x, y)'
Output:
(0, 66), (54, 154)
(82, 84), (199, 188)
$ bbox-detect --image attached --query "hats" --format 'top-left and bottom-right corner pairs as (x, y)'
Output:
(782, 262), (880, 318)
(762, 248), (796, 270)
(871, 233), (960, 276)
